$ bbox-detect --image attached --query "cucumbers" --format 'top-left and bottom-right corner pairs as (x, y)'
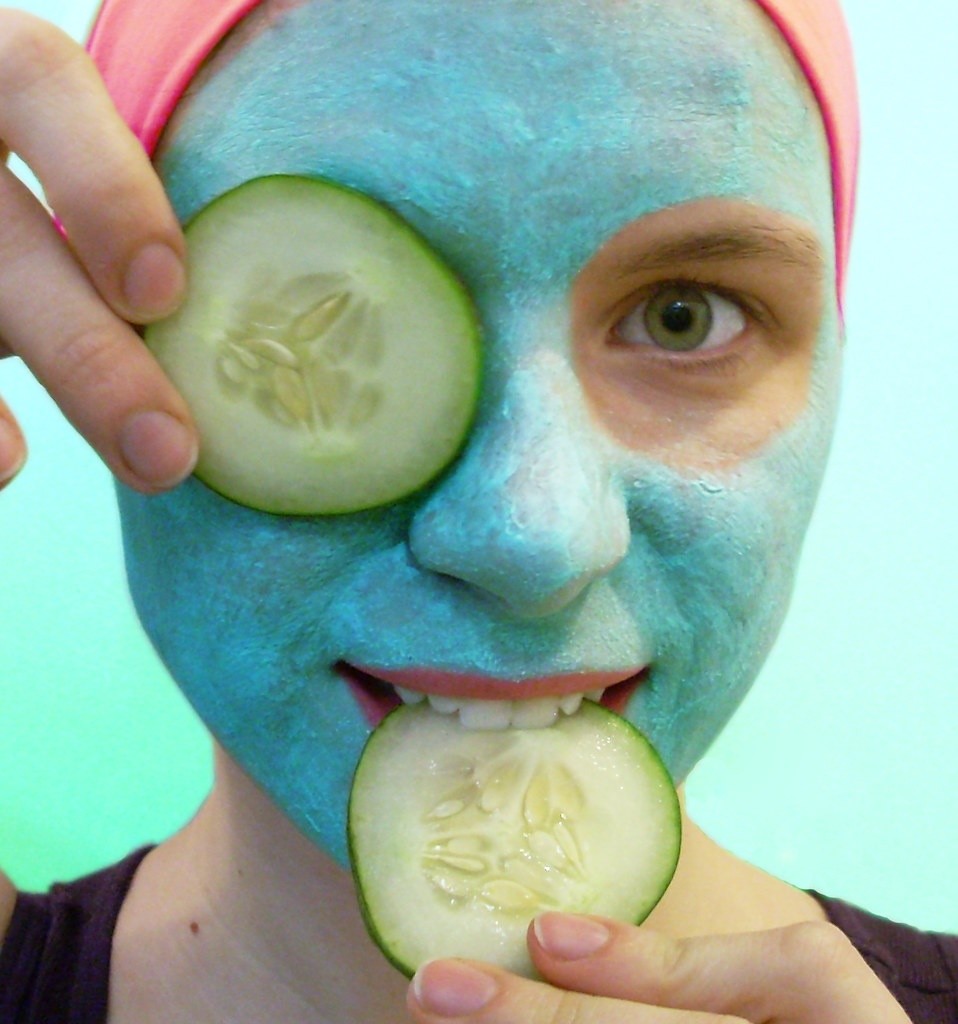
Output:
(348, 692), (682, 981)
(140, 174), (484, 514)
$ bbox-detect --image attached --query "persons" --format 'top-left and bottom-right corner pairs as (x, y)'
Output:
(0, 0), (958, 1021)
